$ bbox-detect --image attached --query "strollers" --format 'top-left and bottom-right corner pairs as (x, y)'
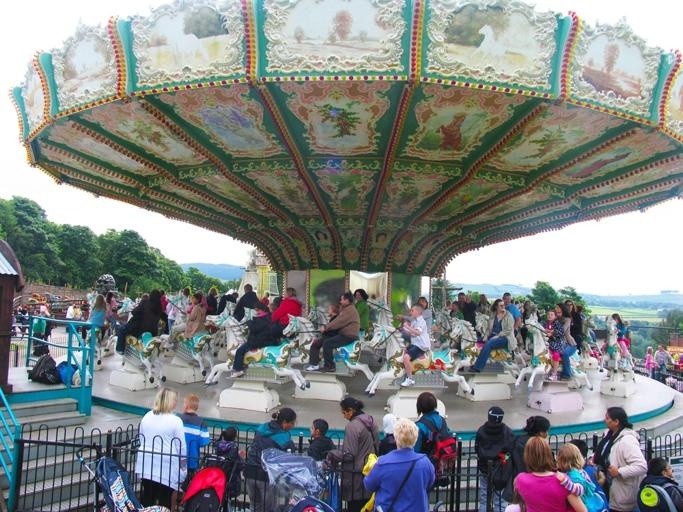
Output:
(75, 445), (170, 511)
(176, 452), (239, 511)
(260, 446), (337, 510)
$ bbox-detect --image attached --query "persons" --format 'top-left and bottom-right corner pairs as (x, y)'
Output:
(396, 296), (431, 386)
(16, 296), (61, 356)
(134, 392), (683, 511)
(116, 284), (302, 376)
(443, 292), (597, 381)
(646, 343), (682, 382)
(307, 289), (369, 372)
(66, 293), (117, 340)
(600, 314), (633, 368)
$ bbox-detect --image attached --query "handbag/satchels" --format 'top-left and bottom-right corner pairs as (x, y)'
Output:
(492, 452), (513, 490)
(41, 368), (60, 384)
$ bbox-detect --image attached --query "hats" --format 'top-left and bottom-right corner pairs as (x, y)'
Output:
(488, 407), (504, 423)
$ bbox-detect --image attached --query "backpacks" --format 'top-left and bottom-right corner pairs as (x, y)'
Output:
(636, 482), (678, 512)
(421, 416), (458, 487)
(27, 354), (56, 381)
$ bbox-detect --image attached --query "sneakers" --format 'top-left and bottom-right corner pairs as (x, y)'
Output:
(307, 364), (321, 371)
(322, 366), (334, 370)
(401, 377), (416, 387)
(231, 370), (244, 378)
